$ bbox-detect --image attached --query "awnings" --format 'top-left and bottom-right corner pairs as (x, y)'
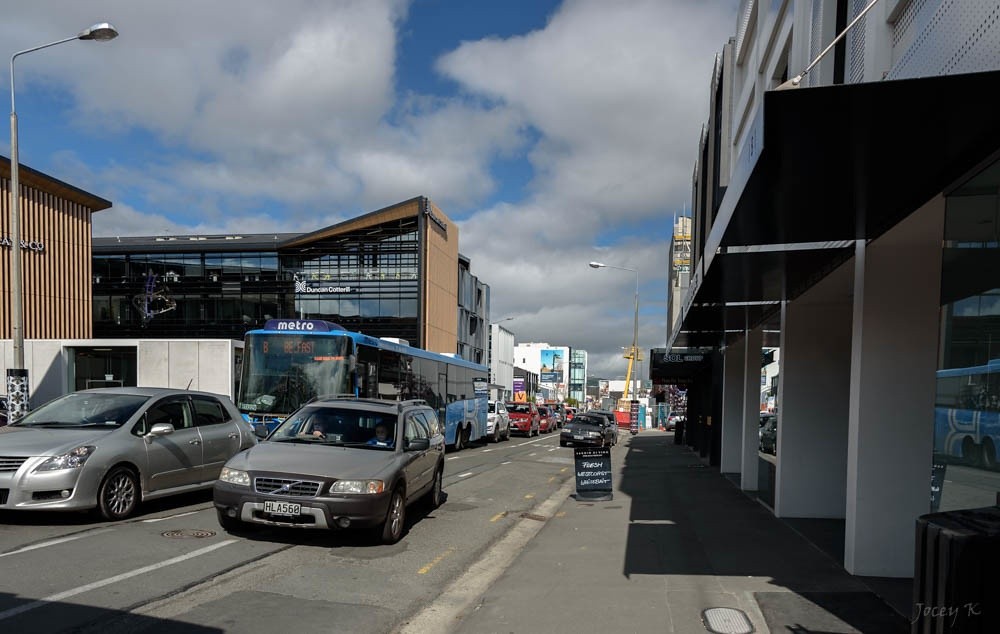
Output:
(667, 68), (1000, 358)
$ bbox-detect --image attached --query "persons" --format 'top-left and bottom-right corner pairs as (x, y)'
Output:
(299, 415), (329, 441)
(367, 421), (395, 448)
(86, 399), (123, 425)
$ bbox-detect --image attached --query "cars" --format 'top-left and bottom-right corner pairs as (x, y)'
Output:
(759, 412), (778, 452)
(664, 410), (687, 431)
(537, 406), (558, 434)
(0, 387), (258, 521)
(560, 412), (616, 451)
(542, 403), (620, 444)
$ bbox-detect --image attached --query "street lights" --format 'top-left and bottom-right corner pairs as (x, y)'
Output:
(485, 316), (515, 400)
(589, 261), (640, 435)
(7, 20), (119, 425)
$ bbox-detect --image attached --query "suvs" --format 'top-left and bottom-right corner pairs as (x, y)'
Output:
(213, 393), (446, 544)
(506, 401), (541, 438)
(236, 317), (490, 451)
(487, 401), (510, 443)
